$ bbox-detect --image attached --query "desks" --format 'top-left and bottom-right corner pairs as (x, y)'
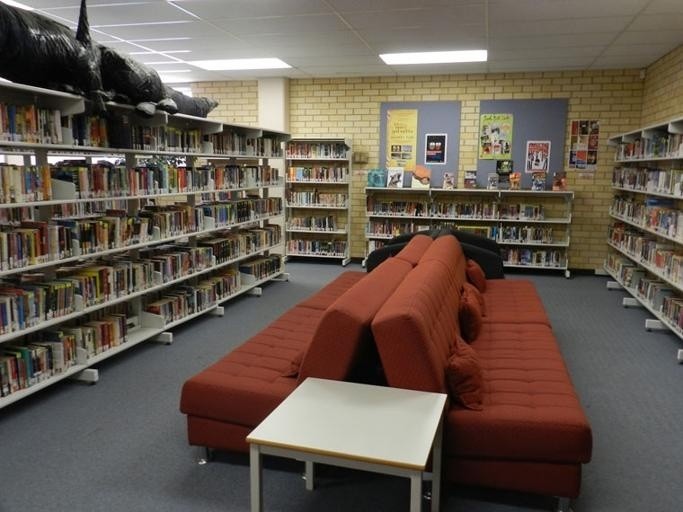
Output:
(243, 377), (450, 511)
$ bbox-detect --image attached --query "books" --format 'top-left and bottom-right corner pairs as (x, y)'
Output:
(1, 102), (283, 397)
(606, 134), (683, 334)
(285, 141), (347, 256)
(367, 166), (567, 267)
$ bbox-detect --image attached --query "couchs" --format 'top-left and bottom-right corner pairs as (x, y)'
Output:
(178, 234), (591, 511)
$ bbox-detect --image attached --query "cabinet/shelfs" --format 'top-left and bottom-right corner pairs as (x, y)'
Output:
(361, 186), (574, 278)
(2, 79), (291, 406)
(595, 118), (683, 363)
(284, 137), (354, 266)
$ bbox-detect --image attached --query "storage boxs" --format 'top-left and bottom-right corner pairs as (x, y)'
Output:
(387, 167), (413, 188)
(367, 168), (387, 187)
